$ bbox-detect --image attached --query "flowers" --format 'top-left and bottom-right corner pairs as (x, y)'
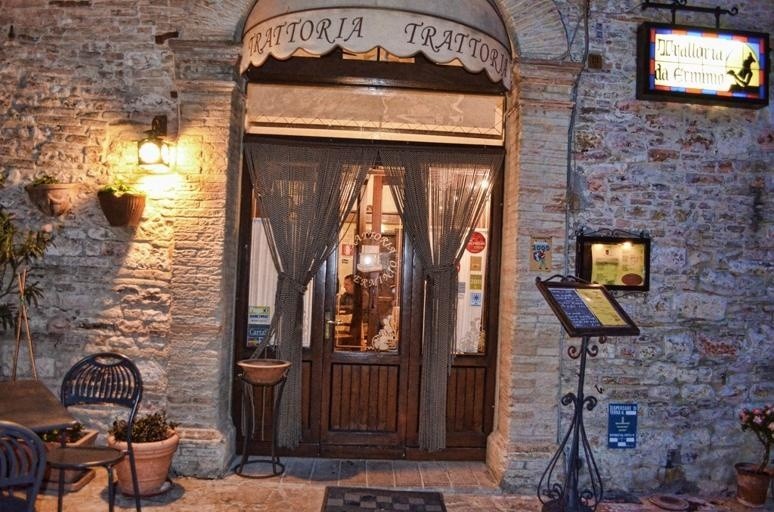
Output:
(736, 403), (774, 475)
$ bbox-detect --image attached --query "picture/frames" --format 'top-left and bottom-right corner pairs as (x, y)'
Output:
(573, 228), (652, 293)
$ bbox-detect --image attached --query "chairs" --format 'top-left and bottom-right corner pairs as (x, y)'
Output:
(45, 353), (144, 512)
(0, 420), (48, 512)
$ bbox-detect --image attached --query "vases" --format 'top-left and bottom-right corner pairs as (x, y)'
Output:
(731, 462), (773, 509)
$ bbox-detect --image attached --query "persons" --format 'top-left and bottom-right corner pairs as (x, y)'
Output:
(340, 274), (370, 313)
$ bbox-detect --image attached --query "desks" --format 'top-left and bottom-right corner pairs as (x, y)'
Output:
(0, 378), (76, 511)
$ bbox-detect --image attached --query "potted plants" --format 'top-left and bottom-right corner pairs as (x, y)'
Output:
(102, 406), (182, 499)
(24, 174), (81, 220)
(35, 422), (98, 494)
(98, 171), (148, 230)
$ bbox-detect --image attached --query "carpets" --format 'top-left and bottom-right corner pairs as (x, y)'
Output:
(318, 483), (446, 512)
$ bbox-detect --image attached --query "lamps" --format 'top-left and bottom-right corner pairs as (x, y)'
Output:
(136, 110), (182, 171)
